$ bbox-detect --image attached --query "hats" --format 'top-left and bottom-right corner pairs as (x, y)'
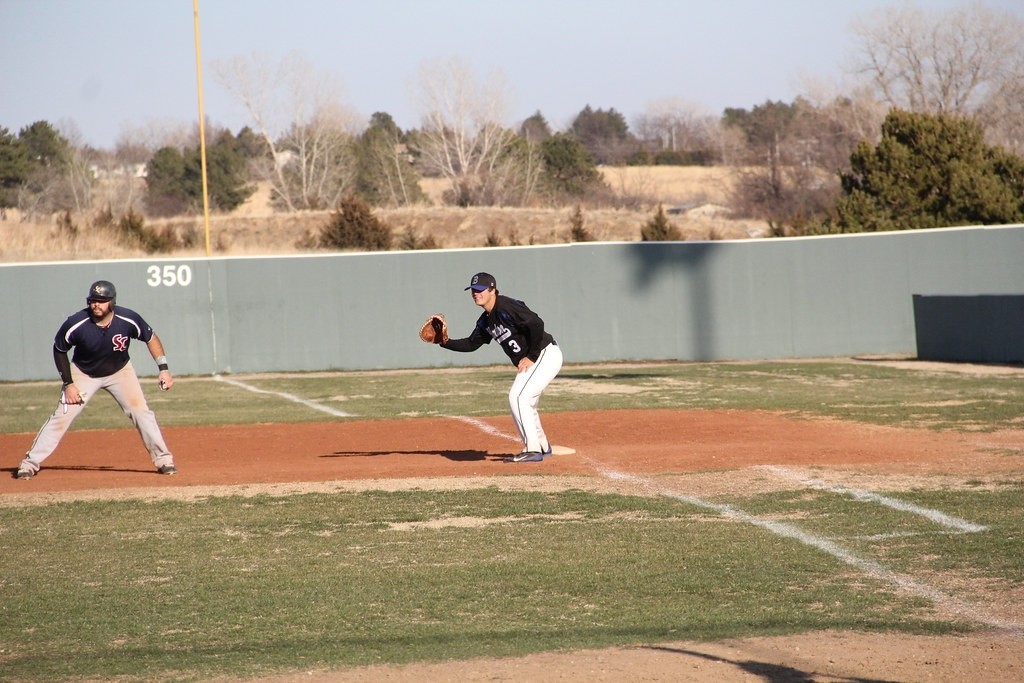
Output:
(463, 272), (496, 291)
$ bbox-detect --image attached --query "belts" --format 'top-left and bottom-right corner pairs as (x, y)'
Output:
(552, 340), (558, 345)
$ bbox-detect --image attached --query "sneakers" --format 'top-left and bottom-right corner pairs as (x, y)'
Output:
(542, 443), (552, 456)
(158, 465), (176, 475)
(503, 450), (543, 462)
(16, 466), (34, 480)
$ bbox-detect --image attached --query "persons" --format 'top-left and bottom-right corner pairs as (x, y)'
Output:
(16, 281), (178, 478)
(419, 272), (563, 462)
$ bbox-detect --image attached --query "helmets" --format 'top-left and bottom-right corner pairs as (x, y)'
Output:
(87, 280), (116, 311)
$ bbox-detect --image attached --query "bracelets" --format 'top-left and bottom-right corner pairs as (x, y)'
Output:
(158, 363), (168, 371)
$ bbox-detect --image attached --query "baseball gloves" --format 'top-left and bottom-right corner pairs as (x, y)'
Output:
(418, 312), (450, 345)
